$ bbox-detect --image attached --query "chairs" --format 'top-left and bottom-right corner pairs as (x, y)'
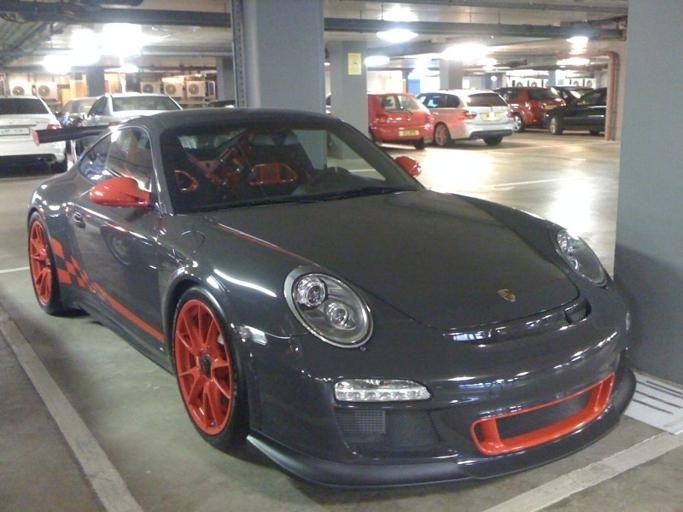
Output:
(141, 130), (222, 197)
(239, 128), (315, 194)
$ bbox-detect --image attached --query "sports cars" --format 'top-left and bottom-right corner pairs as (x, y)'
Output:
(16, 103), (640, 499)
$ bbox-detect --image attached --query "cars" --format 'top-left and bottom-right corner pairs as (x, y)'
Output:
(74, 92), (197, 166)
(491, 83), (567, 131)
(367, 92), (435, 150)
(52, 97), (98, 134)
(204, 100), (236, 109)
(552, 83), (596, 105)
(541, 85), (606, 137)
(0, 94), (68, 174)
(414, 88), (515, 147)
